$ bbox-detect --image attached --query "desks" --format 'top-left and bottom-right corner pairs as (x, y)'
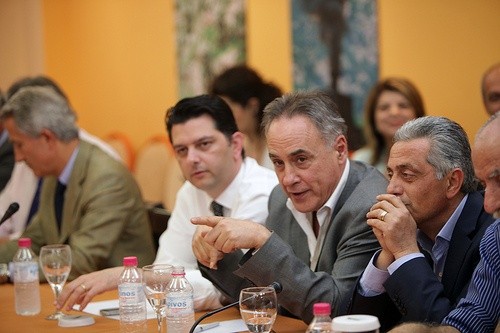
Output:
(0, 278), (308, 333)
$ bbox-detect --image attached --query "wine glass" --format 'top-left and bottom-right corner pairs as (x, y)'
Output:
(39, 244), (81, 321)
(141, 263), (176, 333)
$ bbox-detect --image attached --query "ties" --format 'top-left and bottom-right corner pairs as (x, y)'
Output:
(209, 200), (225, 218)
(52, 178), (67, 235)
(25, 177), (43, 227)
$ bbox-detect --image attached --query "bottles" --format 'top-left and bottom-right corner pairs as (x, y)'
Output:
(117, 256), (149, 333)
(13, 237), (41, 316)
(164, 264), (196, 333)
(305, 302), (333, 333)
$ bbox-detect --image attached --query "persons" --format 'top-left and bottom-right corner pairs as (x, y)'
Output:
(0, 76), (156, 281)
(482, 63), (500, 114)
(325, 91), (364, 158)
(353, 77), (427, 170)
(347, 115), (495, 332)
(54, 94), (278, 311)
(388, 112), (500, 333)
(211, 66), (283, 168)
(191, 91), (391, 326)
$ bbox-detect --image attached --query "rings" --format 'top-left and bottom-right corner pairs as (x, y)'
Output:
(381, 211), (387, 221)
(80, 284), (87, 291)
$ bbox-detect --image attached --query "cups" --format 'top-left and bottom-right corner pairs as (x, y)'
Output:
(239, 286), (278, 333)
(331, 314), (381, 333)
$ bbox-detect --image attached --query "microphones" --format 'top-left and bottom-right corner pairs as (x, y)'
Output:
(190, 281), (283, 333)
(0, 202), (20, 226)
